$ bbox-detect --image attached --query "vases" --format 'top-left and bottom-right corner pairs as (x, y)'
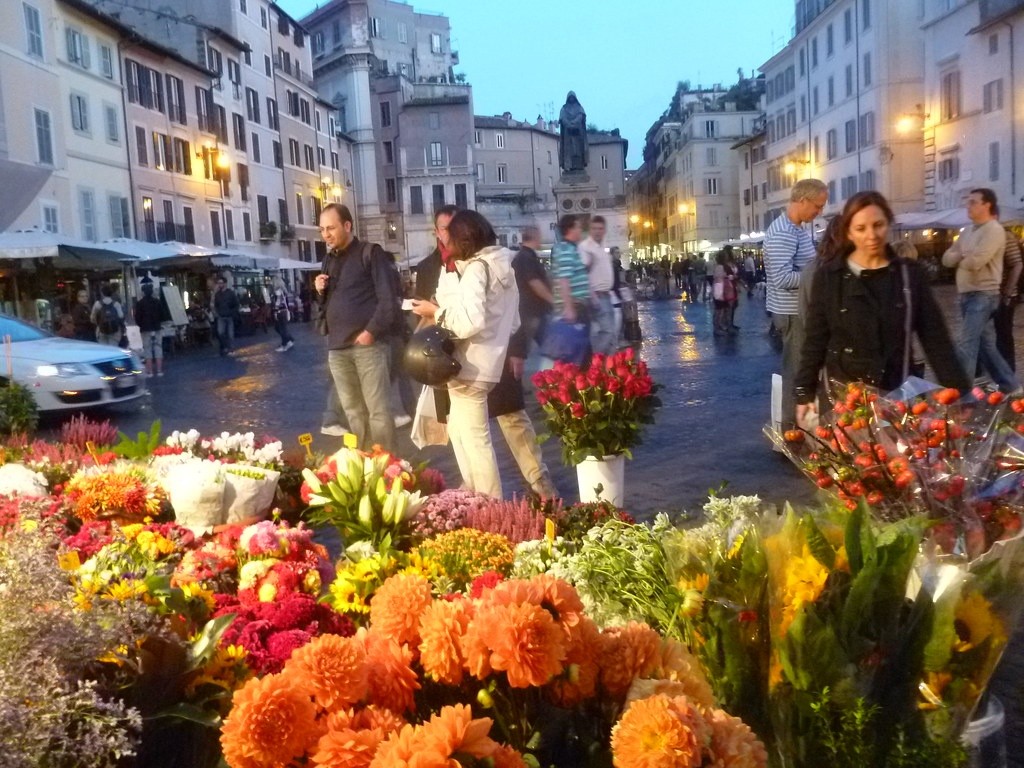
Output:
(570, 448), (625, 510)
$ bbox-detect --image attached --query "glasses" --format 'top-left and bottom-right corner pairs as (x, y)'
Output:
(805, 197), (825, 213)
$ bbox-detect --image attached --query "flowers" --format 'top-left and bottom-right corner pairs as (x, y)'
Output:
(2, 342), (1024, 767)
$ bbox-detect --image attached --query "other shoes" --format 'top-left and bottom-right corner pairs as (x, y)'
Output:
(157, 369), (169, 377)
(222, 351), (234, 357)
(986, 382), (999, 392)
(1000, 387), (1024, 402)
(320, 425), (348, 436)
(283, 341), (293, 351)
(974, 377), (992, 386)
(147, 370), (154, 378)
(714, 326), (730, 336)
(392, 416), (411, 427)
(275, 345), (285, 352)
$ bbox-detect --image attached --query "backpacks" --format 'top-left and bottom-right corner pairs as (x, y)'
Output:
(95, 299), (119, 335)
(363, 241), (402, 337)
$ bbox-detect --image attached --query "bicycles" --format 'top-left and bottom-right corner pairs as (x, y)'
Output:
(626, 275), (662, 300)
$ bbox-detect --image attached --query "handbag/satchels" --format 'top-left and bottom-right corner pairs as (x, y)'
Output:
(410, 384), (449, 451)
(539, 318), (588, 366)
(826, 378), (900, 411)
(714, 282), (736, 302)
(286, 310), (291, 321)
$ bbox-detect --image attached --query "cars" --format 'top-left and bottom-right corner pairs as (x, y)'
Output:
(0, 315), (151, 431)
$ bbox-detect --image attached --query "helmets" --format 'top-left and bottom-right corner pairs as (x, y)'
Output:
(403, 326), (462, 385)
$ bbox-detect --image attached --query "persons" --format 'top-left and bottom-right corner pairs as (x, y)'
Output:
(73, 283), (124, 347)
(942, 188), (1024, 403)
(134, 284), (166, 378)
(206, 276), (240, 357)
(762, 179), (828, 453)
(270, 277), (295, 353)
(792, 190), (972, 429)
(315, 203), (636, 515)
(636, 244), (756, 335)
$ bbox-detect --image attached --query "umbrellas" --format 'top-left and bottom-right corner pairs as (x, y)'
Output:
(0, 223), (321, 280)
